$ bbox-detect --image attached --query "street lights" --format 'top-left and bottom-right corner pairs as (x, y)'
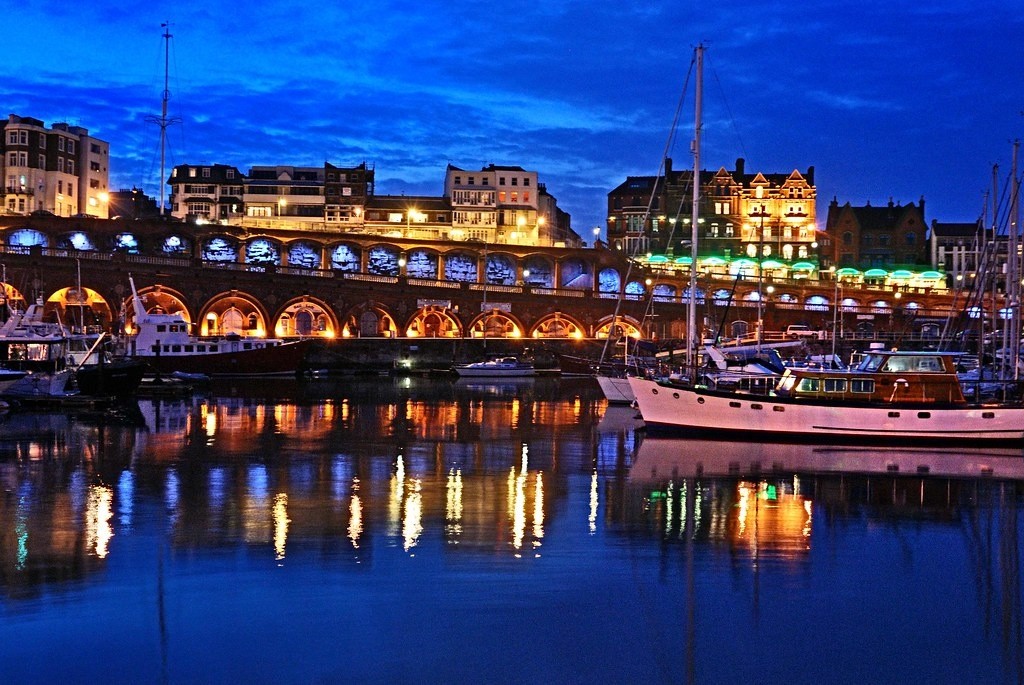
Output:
(277, 199), (287, 219)
(407, 209), (418, 236)
(516, 218), (524, 247)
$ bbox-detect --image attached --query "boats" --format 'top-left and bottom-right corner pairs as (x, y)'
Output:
(60, 272), (319, 405)
(584, 44), (1024, 443)
(447, 356), (562, 378)
(0, 255), (149, 401)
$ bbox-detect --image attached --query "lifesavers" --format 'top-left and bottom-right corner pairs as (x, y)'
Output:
(893, 378), (910, 393)
(256, 343), (263, 349)
